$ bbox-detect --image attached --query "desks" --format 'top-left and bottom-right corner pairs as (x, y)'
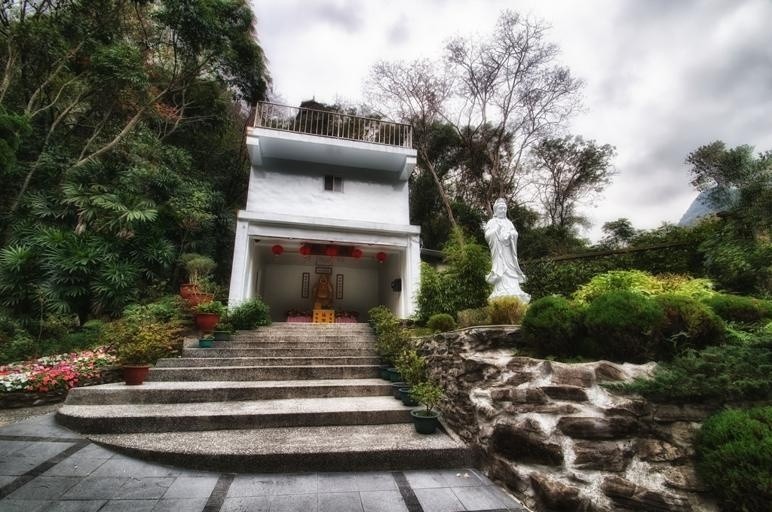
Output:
(286, 314), (312, 323)
(312, 309), (336, 325)
(334, 315), (358, 323)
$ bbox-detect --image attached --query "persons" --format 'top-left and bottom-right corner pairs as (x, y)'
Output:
(312, 274), (334, 307)
(483, 198), (531, 300)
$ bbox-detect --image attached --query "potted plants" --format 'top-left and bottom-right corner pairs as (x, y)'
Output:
(203, 280), (218, 303)
(199, 331), (216, 347)
(367, 304), (444, 433)
(177, 254), (218, 298)
(101, 309), (165, 386)
(187, 278), (210, 306)
(196, 297), (218, 331)
(213, 310), (237, 341)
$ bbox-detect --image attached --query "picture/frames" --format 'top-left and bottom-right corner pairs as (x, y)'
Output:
(335, 273), (346, 299)
(301, 271), (311, 298)
(314, 264), (334, 275)
